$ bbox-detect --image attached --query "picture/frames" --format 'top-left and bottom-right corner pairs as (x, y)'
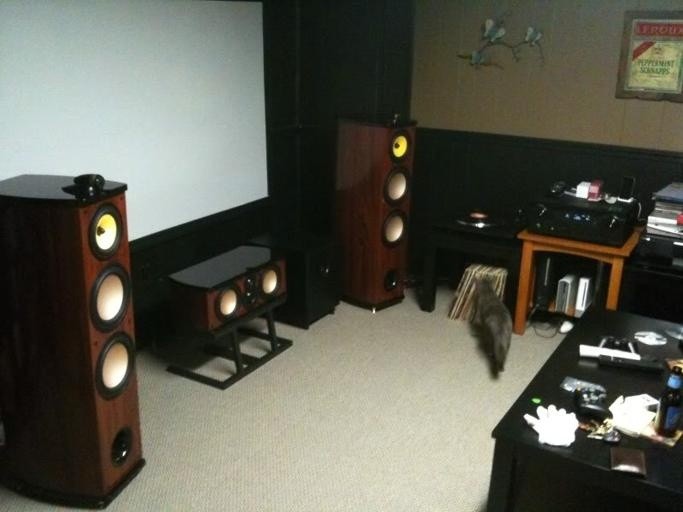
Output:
(614, 9), (683, 104)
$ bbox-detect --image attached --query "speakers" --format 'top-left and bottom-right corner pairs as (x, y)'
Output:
(167, 247), (289, 331)
(333, 119), (418, 312)
(1, 173), (147, 508)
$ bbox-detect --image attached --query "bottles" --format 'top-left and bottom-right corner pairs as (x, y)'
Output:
(654, 365), (683, 437)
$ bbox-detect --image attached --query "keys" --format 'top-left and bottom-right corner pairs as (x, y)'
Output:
(586, 417), (622, 445)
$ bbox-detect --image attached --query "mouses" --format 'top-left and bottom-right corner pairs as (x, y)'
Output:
(73, 176), (106, 195)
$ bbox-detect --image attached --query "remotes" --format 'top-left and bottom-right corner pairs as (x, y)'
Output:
(602, 353), (666, 373)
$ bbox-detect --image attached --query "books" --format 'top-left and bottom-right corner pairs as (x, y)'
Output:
(449, 262), (509, 319)
(646, 182), (683, 241)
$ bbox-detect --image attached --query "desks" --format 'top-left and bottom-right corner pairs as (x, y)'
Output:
(486, 301), (683, 512)
(512, 229), (642, 335)
(165, 295), (295, 390)
(417, 210), (519, 313)
(615, 237), (683, 320)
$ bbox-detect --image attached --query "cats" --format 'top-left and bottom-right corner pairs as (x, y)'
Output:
(468, 276), (512, 379)
